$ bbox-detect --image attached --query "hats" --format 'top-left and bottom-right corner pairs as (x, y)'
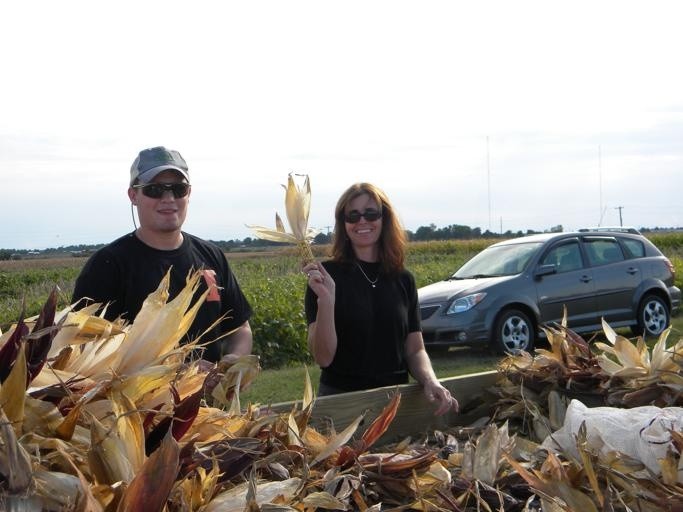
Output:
(131, 147), (188, 185)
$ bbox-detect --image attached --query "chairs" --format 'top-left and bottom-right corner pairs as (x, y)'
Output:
(546, 248), (624, 270)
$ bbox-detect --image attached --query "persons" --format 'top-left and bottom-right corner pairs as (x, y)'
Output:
(69, 147), (252, 458)
(299, 182), (461, 417)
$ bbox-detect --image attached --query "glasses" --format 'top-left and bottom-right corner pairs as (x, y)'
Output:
(341, 211), (381, 223)
(131, 183), (190, 198)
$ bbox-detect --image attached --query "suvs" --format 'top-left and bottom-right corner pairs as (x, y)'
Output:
(405, 228), (680, 361)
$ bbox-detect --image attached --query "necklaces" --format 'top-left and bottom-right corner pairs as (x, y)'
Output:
(357, 261), (383, 288)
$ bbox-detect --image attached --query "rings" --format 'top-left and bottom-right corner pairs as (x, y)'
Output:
(306, 272), (311, 278)
